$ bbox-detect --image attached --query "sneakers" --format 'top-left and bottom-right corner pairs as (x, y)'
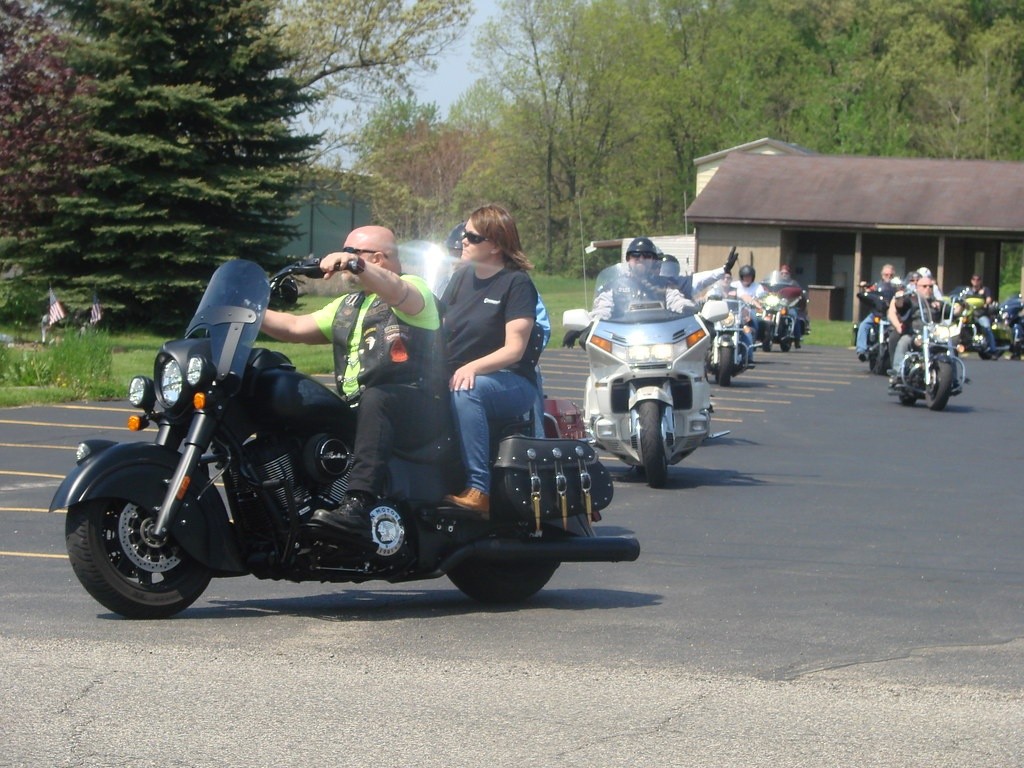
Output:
(442, 487), (490, 514)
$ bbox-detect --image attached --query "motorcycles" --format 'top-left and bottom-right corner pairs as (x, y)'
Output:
(563, 260), (730, 489)
(890, 277), (969, 411)
(49, 259), (640, 619)
(757, 269), (811, 352)
(853, 278), (895, 375)
(992, 293), (1024, 361)
(696, 283), (762, 387)
(948, 286), (1006, 360)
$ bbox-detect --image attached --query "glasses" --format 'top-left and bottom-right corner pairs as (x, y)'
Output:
(461, 228), (489, 244)
(630, 252), (654, 259)
(917, 285), (933, 288)
(342, 247), (389, 259)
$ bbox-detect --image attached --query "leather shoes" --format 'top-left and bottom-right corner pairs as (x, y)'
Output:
(311, 496), (373, 540)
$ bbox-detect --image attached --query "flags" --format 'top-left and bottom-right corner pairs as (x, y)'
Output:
(90, 283), (101, 327)
(49, 287), (65, 327)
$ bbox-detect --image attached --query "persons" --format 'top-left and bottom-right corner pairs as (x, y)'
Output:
(760, 265), (806, 348)
(963, 272), (998, 360)
(730, 264), (766, 369)
(245, 225), (450, 540)
(562, 236), (738, 413)
(855, 264), (970, 396)
(438, 205), (551, 520)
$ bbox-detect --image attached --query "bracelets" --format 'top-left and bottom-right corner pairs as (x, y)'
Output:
(352, 256), (364, 275)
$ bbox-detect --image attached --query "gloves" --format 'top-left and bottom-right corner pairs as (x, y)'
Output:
(724, 246), (739, 272)
(562, 330), (581, 349)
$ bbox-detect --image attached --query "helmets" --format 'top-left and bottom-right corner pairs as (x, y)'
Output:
(446, 223), (466, 248)
(625, 237), (657, 263)
(739, 265), (755, 282)
